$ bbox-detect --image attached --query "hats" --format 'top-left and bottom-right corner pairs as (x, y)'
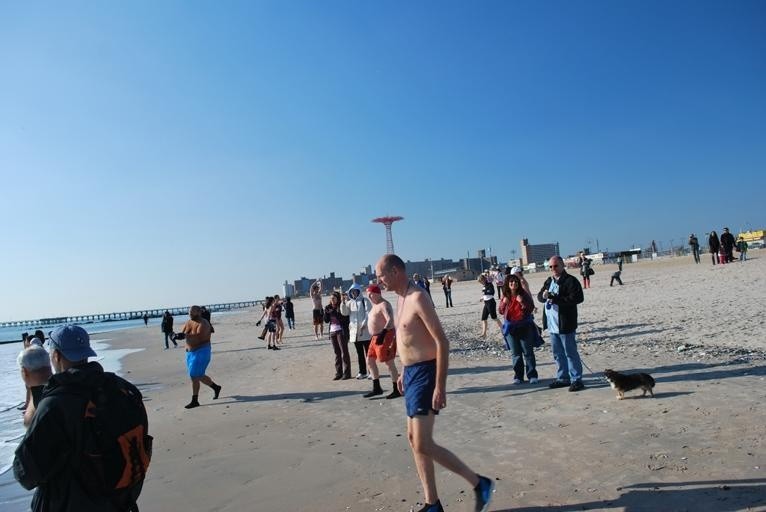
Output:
(509, 267), (522, 276)
(47, 324), (98, 361)
(366, 285), (381, 294)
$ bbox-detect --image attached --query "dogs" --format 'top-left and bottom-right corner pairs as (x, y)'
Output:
(603, 368), (656, 400)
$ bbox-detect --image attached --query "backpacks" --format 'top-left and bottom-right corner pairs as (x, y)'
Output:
(71, 372), (154, 509)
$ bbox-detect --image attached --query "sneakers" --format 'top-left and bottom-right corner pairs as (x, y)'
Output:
(386, 391), (401, 399)
(472, 474), (496, 512)
(529, 377), (538, 385)
(569, 382), (584, 392)
(418, 498), (444, 512)
(363, 389), (383, 398)
(332, 371), (372, 380)
(549, 381), (570, 388)
(512, 379), (524, 384)
(257, 336), (280, 350)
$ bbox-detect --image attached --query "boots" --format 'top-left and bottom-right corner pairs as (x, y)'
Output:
(210, 382), (222, 399)
(184, 394), (200, 409)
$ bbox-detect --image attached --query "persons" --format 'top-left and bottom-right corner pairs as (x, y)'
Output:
(339, 283), (372, 380)
(161, 312), (178, 351)
(482, 264), (512, 300)
(579, 252), (590, 288)
(610, 270), (622, 286)
(720, 243), (726, 264)
(16, 329), (53, 427)
(617, 256), (622, 271)
(736, 238), (748, 261)
(537, 255), (585, 391)
(141, 314), (151, 327)
(709, 230), (720, 265)
(309, 278), (326, 340)
(13, 323), (153, 511)
(255, 295), (295, 350)
(499, 274), (545, 384)
(200, 307), (211, 345)
(510, 266), (546, 352)
(413, 273), (424, 288)
(424, 277), (435, 308)
(324, 290), (352, 380)
(362, 285), (400, 399)
(478, 275), (502, 338)
(376, 254), (495, 512)
(721, 228), (735, 262)
(173, 306), (221, 409)
(442, 273), (453, 308)
(689, 234), (700, 264)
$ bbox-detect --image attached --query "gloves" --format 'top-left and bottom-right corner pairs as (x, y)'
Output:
(171, 332), (185, 341)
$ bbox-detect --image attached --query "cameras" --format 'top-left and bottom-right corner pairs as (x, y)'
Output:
(546, 291), (557, 299)
